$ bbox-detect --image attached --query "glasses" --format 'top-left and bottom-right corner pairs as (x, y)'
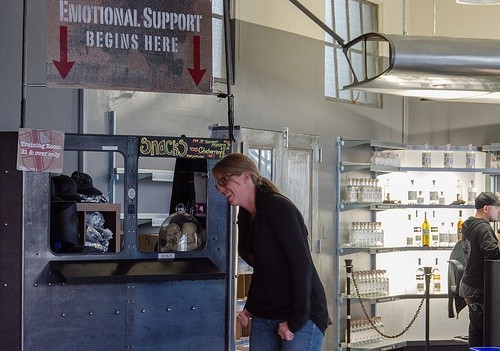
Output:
(215, 174), (234, 192)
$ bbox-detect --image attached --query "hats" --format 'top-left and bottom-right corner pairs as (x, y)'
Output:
(475, 192), (500, 209)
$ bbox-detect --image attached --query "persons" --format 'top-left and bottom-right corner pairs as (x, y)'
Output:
(211, 153), (332, 351)
(461, 190), (500, 351)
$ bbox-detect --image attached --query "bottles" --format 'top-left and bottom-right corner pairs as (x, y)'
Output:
(421, 142), (431, 168)
(466, 144), (475, 168)
(345, 315), (384, 348)
(344, 178), (383, 204)
(432, 258), (441, 293)
(449, 222), (457, 247)
(349, 221), (384, 247)
(384, 179), (476, 206)
(416, 258), (425, 294)
(414, 210), (422, 247)
(407, 214), (413, 246)
(444, 144), (453, 168)
(439, 222), (449, 247)
(431, 210), (439, 247)
(422, 212), (429, 247)
(350, 270), (389, 298)
(369, 151), (401, 168)
(457, 210), (464, 242)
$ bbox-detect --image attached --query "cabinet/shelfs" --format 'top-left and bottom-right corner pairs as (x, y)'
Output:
(334, 135), (500, 351)
(209, 124), (323, 351)
(0, 130), (232, 351)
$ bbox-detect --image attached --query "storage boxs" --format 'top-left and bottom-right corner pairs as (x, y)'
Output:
(76, 203), (121, 253)
